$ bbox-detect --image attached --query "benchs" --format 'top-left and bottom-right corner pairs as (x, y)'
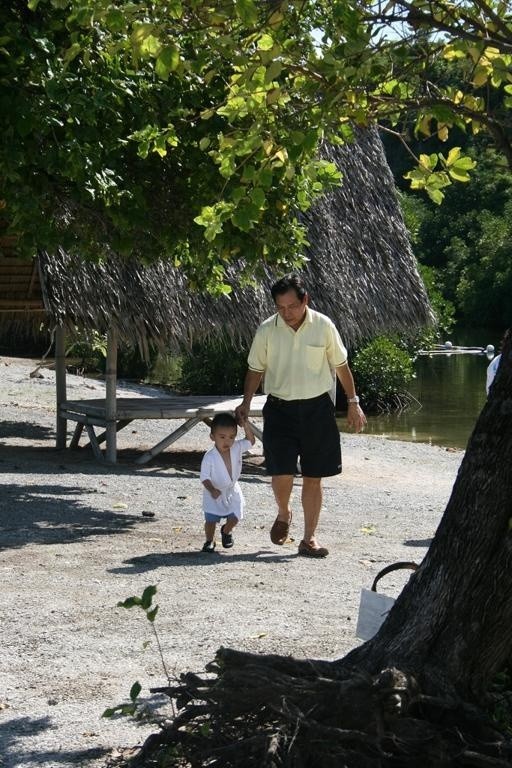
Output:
(58, 393), (267, 465)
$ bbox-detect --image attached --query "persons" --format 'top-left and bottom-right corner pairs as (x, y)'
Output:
(196, 411), (256, 552)
(234, 273), (367, 556)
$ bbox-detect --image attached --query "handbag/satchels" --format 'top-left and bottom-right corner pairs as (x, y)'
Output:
(355, 562), (418, 640)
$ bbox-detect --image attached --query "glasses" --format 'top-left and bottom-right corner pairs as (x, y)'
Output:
(277, 303), (298, 311)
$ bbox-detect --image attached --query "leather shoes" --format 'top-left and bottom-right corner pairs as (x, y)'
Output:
(270, 510), (292, 545)
(221, 525), (234, 548)
(298, 539), (328, 558)
(202, 540), (216, 551)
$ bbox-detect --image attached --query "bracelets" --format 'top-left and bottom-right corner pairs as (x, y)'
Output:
(346, 395), (360, 403)
